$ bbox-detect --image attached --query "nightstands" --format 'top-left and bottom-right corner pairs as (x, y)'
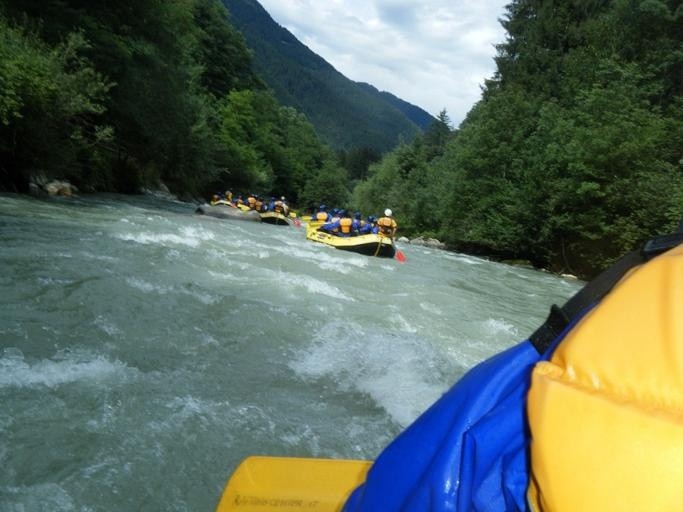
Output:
(309, 221), (330, 227)
(217, 456), (376, 511)
(390, 236), (406, 261)
(302, 216), (313, 221)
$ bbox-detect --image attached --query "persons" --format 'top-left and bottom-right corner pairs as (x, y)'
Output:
(309, 203), (397, 239)
(210, 187), (303, 225)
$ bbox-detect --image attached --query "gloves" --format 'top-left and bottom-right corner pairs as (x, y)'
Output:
(211, 200), (396, 258)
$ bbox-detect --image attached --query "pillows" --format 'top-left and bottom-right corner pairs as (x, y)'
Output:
(319, 204), (395, 225)
(216, 187), (287, 202)
